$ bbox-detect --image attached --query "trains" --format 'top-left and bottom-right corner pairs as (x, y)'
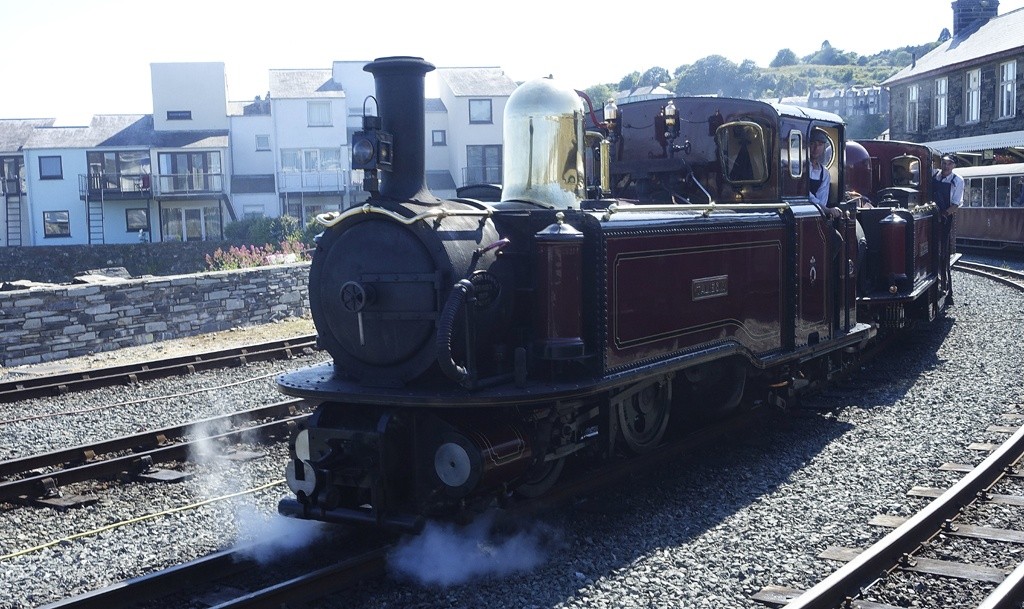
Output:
(274, 53), (963, 540)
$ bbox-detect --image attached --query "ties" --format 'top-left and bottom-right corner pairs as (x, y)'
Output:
(939, 176), (946, 182)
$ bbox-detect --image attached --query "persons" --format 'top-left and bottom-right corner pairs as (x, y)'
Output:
(807, 130), (843, 218)
(933, 153), (964, 235)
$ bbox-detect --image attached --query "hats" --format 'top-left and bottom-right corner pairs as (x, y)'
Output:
(810, 130), (827, 143)
(941, 152), (959, 165)
